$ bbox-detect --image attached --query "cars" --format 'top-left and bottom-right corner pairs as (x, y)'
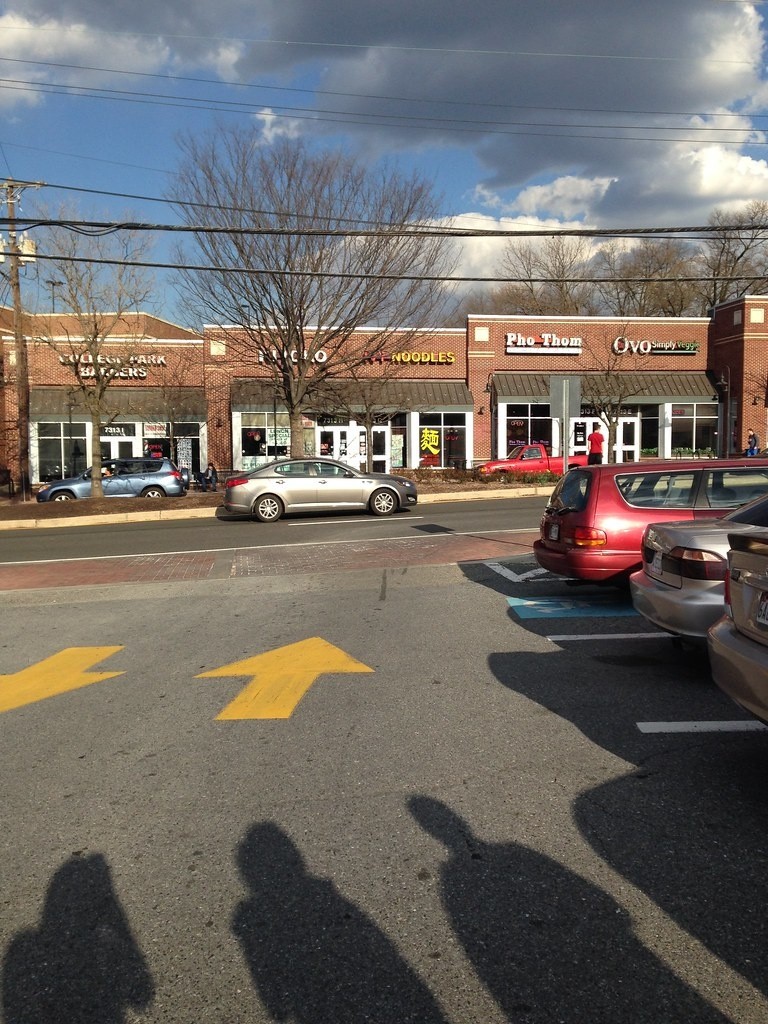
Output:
(533, 457), (768, 582)
(630, 493), (767, 647)
(223, 456), (418, 523)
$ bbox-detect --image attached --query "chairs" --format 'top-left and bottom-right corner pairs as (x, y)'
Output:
(715, 486), (737, 508)
(748, 490), (767, 499)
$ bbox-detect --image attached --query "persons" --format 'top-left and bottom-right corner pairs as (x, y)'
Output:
(747, 428), (759, 457)
(587, 424), (604, 465)
(201, 463), (219, 493)
(103, 466), (112, 477)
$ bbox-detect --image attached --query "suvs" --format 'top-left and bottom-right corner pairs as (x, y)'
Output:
(36, 456), (190, 503)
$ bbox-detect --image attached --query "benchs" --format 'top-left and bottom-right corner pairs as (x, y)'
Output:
(194, 469), (239, 490)
(0, 469), (15, 499)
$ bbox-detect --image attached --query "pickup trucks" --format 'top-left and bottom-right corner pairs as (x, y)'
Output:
(476, 444), (590, 483)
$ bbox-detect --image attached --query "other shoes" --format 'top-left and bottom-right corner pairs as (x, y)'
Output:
(201, 490), (207, 494)
(212, 490), (218, 493)
